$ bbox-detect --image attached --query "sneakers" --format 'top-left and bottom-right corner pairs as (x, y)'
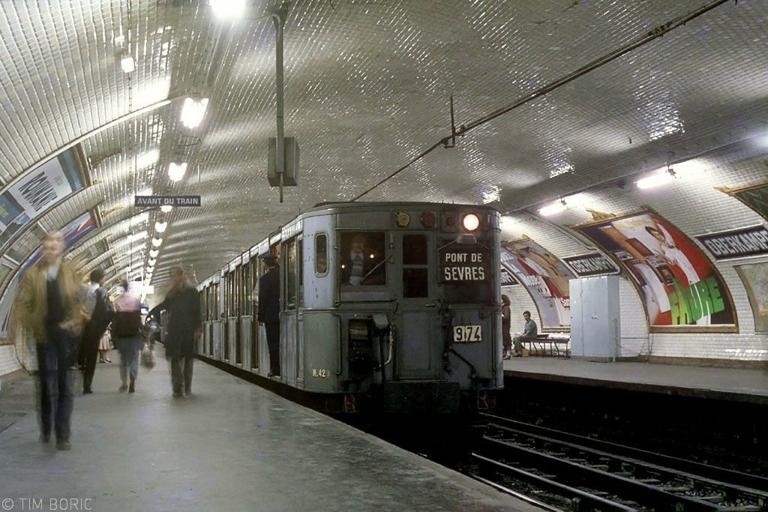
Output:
(55, 437), (71, 449)
(267, 371), (279, 377)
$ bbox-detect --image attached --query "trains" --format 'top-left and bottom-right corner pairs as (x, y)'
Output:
(144, 198), (505, 416)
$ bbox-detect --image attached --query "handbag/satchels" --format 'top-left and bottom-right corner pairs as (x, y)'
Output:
(142, 352), (152, 367)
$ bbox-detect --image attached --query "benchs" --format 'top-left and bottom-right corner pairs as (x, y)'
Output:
(523, 338), (570, 357)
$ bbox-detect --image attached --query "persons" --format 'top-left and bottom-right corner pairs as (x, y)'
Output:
(501, 295), (511, 360)
(75, 269), (147, 394)
(513, 311), (537, 357)
(258, 254), (280, 377)
(163, 267), (202, 398)
(13, 231), (97, 450)
(504, 245), (562, 298)
(342, 235), (373, 286)
(645, 218), (711, 325)
